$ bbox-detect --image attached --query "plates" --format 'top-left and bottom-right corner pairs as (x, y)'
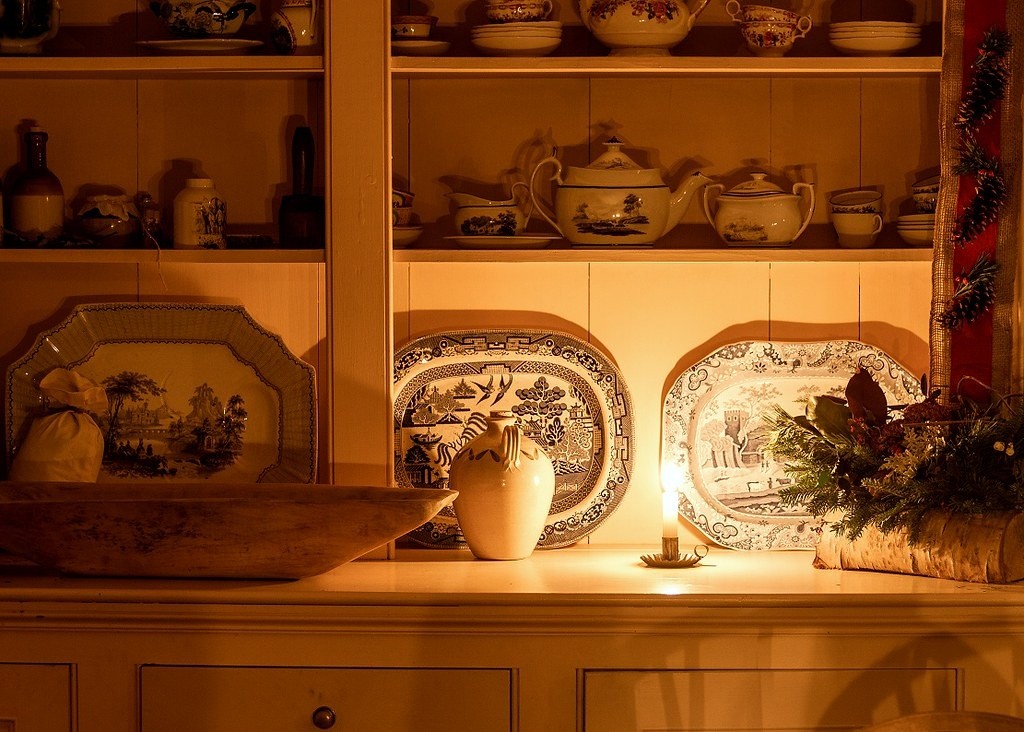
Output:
(133, 38), (264, 57)
(392, 327), (639, 549)
(660, 339), (930, 553)
(391, 40), (451, 57)
(471, 20), (563, 57)
(6, 303), (318, 487)
(442, 232), (563, 250)
(828, 20), (922, 57)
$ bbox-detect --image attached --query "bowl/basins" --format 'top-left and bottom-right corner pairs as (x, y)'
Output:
(390, 16), (439, 39)
(391, 188), (424, 250)
(1, 479), (460, 583)
(159, 0), (256, 38)
(703, 170), (816, 249)
(896, 173), (940, 247)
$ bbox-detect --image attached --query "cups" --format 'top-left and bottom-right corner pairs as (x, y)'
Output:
(724, 0), (812, 56)
(0, 0), (65, 56)
(828, 190), (883, 249)
(578, 0), (710, 59)
(484, 0), (553, 23)
(441, 181), (535, 236)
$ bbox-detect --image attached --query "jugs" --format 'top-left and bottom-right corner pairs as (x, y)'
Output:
(270, 0), (321, 57)
(529, 135), (713, 249)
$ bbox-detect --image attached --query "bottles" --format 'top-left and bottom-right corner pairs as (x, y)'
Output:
(79, 192), (164, 247)
(11, 131), (69, 246)
(172, 177), (227, 250)
(449, 411), (554, 561)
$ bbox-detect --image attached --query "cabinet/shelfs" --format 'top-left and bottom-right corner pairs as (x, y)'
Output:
(389, 0), (978, 563)
(0, 600), (1024, 732)
(0, 0), (327, 590)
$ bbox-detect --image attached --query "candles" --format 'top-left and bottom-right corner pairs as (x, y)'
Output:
(662, 490), (680, 559)
